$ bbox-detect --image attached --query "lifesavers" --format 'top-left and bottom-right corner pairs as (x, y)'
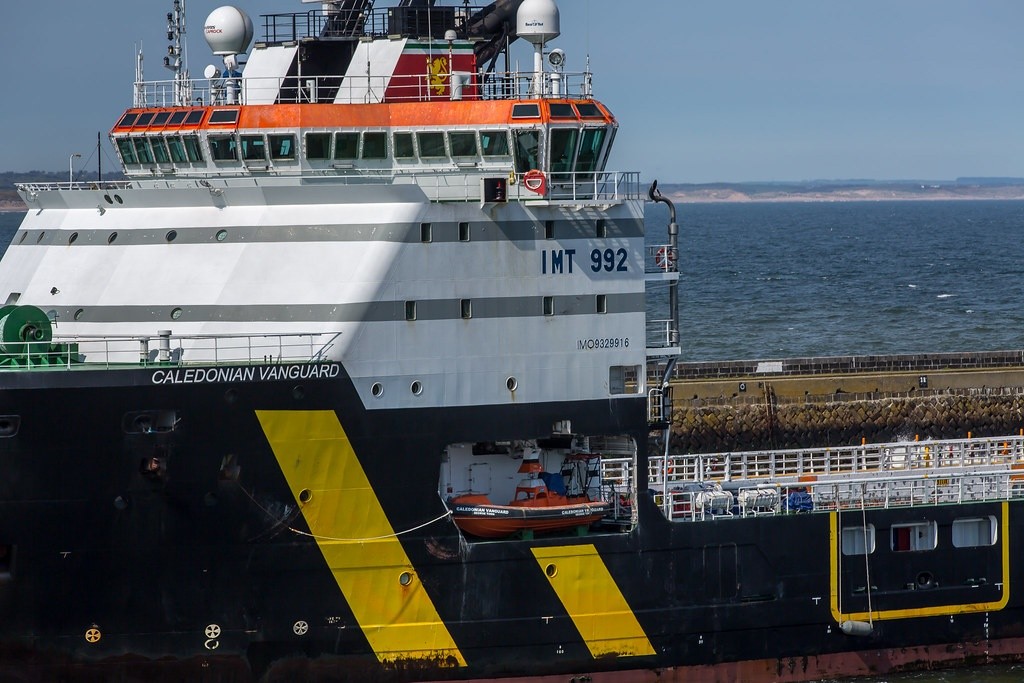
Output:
(527, 169), (544, 177)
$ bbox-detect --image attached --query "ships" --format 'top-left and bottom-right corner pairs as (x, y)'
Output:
(0, 1), (1024, 681)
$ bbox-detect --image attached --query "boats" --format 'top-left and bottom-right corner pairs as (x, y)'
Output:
(449, 463), (606, 536)
(697, 490), (734, 513)
(735, 489), (779, 509)
(659, 493), (698, 517)
